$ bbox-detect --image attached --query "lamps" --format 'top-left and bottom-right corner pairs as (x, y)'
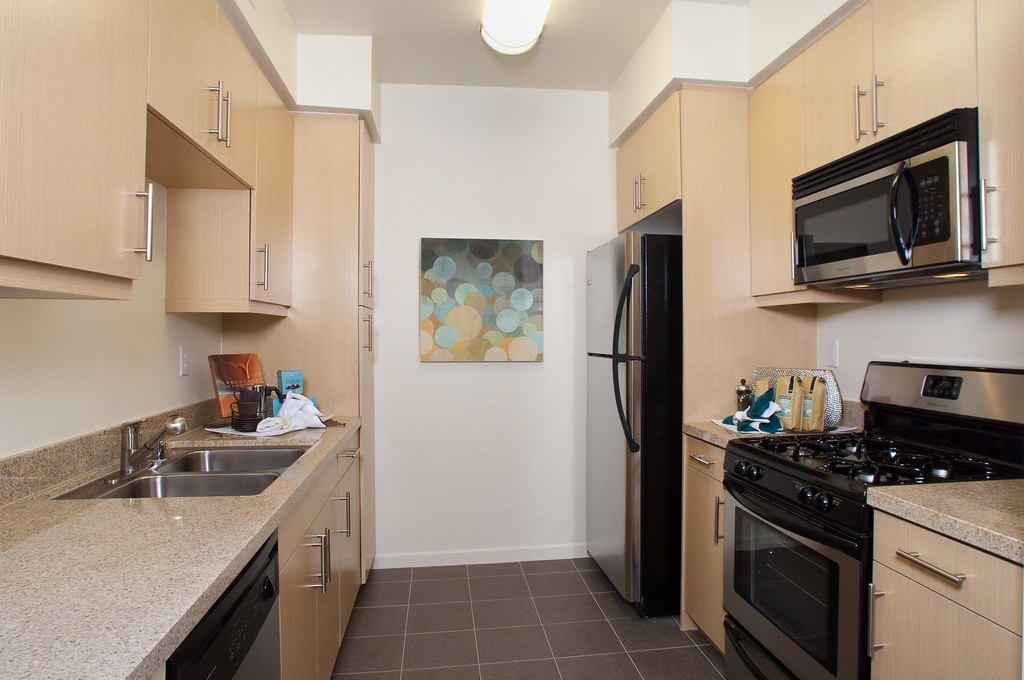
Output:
(480, 0), (553, 56)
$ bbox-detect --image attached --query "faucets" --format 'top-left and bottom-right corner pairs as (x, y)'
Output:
(119, 414), (189, 480)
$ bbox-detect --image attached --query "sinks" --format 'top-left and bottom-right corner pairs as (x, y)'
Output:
(154, 448), (306, 474)
(97, 474), (283, 499)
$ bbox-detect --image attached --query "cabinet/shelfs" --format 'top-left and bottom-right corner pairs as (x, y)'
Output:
(275, 425), (361, 680)
(218, 110), (378, 586)
(613, 85), (681, 237)
(745, 1), (1024, 308)
(0, 0), (292, 319)
(865, 506), (1023, 680)
(675, 432), (730, 657)
